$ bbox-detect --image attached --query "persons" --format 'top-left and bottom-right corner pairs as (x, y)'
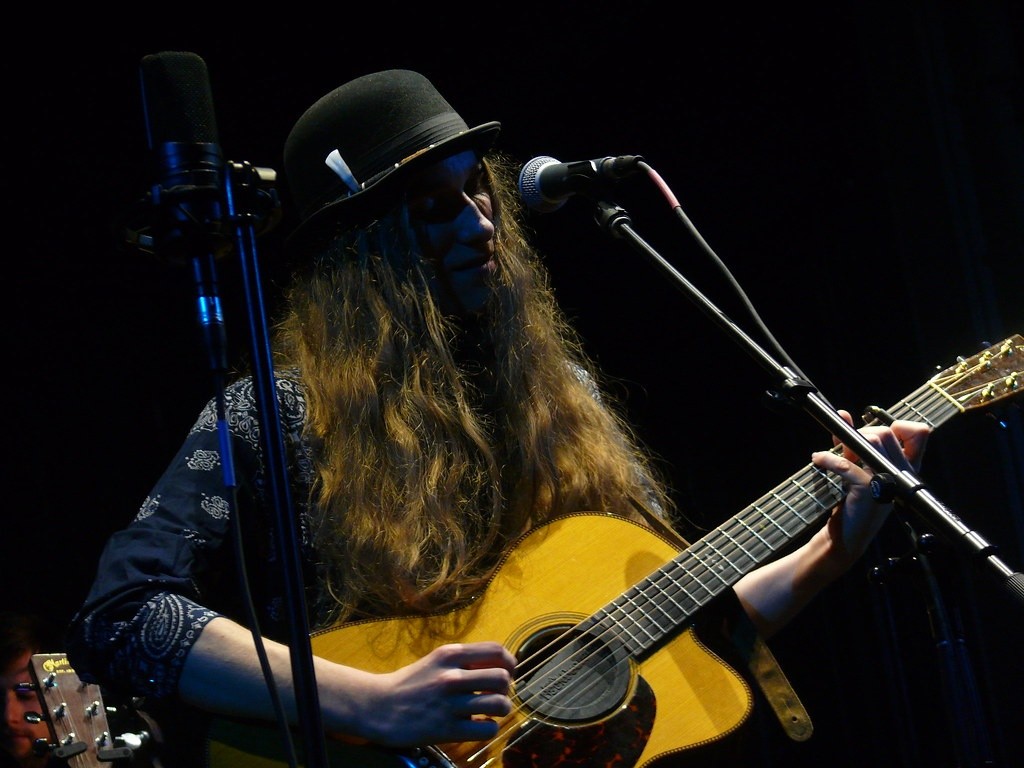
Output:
(70, 70), (928, 768)
(0, 615), (60, 768)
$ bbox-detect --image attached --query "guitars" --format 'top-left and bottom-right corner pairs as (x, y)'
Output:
(17, 652), (134, 768)
(203, 334), (1024, 767)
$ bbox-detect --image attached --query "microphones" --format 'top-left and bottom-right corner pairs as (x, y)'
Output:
(518, 155), (646, 213)
(138, 52), (230, 366)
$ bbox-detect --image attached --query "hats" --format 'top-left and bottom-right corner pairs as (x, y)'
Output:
(275, 67), (501, 249)
